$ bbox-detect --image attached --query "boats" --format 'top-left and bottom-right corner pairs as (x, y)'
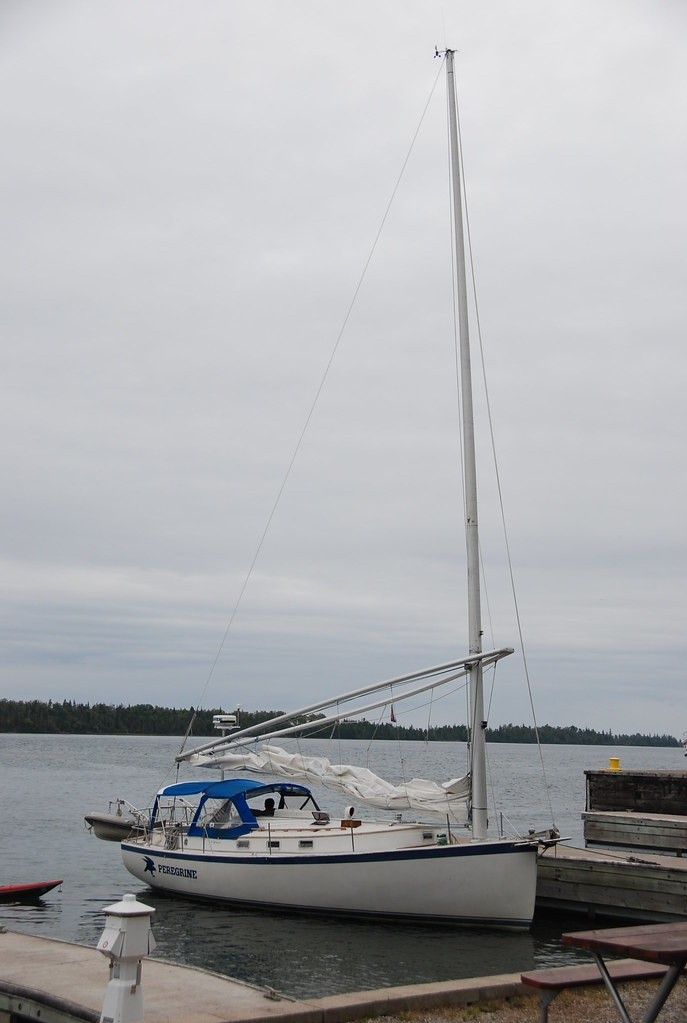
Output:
(0, 880), (65, 901)
(81, 797), (167, 842)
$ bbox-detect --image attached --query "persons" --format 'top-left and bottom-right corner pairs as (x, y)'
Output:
(262, 798), (276, 816)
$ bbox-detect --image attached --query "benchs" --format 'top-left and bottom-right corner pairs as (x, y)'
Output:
(580, 811), (687, 858)
(521, 959), (670, 1023)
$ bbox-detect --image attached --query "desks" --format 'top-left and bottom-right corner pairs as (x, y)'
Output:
(562, 922), (687, 1023)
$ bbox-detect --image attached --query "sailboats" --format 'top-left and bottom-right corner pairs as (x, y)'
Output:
(117, 42), (566, 931)
